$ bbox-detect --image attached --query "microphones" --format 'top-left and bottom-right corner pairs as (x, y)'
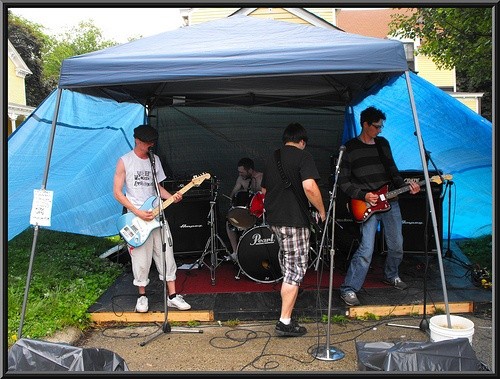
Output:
(148, 149), (154, 167)
(335, 144), (346, 174)
(248, 176), (253, 188)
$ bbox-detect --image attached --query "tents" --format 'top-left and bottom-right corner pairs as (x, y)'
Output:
(18, 13), (452, 338)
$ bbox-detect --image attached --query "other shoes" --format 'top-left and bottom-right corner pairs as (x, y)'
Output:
(224, 252), (236, 262)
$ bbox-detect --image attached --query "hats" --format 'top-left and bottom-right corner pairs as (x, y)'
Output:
(134, 125), (159, 143)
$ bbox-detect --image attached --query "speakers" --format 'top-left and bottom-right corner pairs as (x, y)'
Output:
(160, 194), (218, 253)
(382, 189), (443, 254)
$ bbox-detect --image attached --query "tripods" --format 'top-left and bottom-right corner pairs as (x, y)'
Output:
(140, 158), (204, 347)
(186, 181), (237, 286)
(386, 146), (446, 336)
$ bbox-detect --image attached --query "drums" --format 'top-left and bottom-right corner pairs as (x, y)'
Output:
(227, 189), (258, 230)
(236, 224), (284, 284)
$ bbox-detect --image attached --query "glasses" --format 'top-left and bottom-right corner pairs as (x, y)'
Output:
(368, 123), (384, 130)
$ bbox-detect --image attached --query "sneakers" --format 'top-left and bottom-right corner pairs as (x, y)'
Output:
(167, 294), (191, 310)
(136, 296), (149, 312)
(340, 290), (362, 306)
(274, 317), (307, 336)
(384, 275), (407, 289)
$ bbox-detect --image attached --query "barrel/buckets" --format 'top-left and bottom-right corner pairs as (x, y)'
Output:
(429, 314), (475, 348)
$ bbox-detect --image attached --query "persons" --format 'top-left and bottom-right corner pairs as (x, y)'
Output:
(113, 125), (191, 312)
(338, 107), (421, 306)
(260, 123), (326, 336)
(226, 158), (264, 260)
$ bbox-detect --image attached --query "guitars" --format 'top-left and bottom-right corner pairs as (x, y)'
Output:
(116, 171), (211, 248)
(345, 173), (452, 224)
